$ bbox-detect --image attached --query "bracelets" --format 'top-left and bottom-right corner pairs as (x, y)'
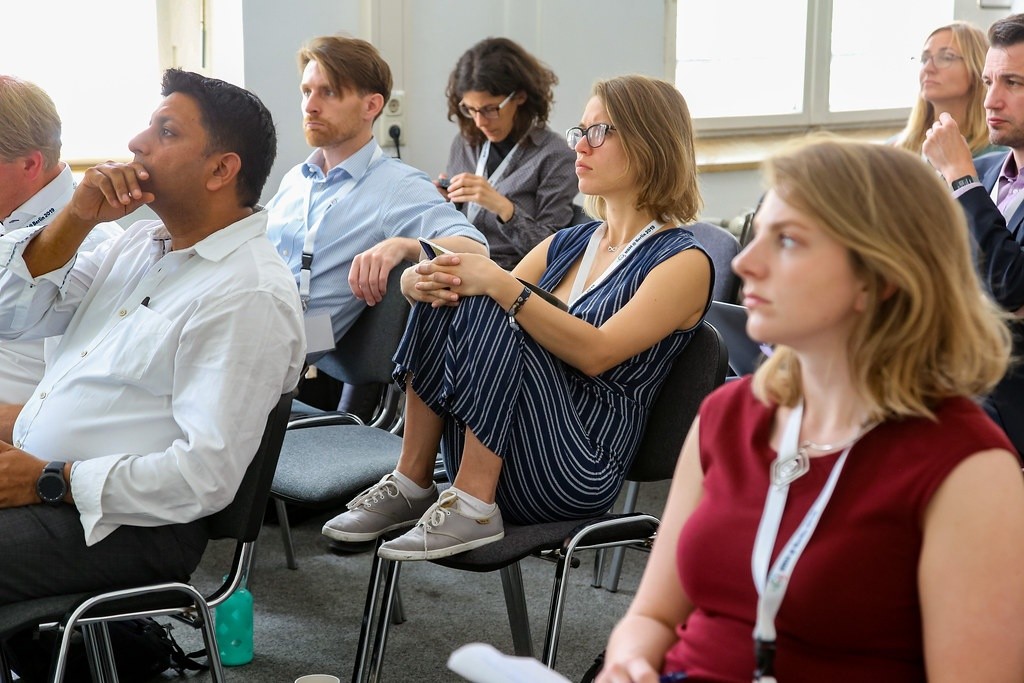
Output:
(508, 286), (532, 317)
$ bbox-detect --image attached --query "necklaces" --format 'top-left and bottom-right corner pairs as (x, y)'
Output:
(607, 234), (629, 252)
(768, 424), (868, 492)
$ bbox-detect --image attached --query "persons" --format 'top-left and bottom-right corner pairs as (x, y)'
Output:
(891, 11), (1024, 466)
(0, 67), (307, 683)
(589, 133), (1024, 683)
(264, 34), (715, 563)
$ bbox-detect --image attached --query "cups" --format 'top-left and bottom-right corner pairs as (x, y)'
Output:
(295, 674), (342, 683)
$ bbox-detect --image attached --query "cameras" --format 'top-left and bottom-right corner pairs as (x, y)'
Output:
(439, 178), (451, 188)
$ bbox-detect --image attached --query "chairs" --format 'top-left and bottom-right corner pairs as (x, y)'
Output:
(33, 216), (782, 683)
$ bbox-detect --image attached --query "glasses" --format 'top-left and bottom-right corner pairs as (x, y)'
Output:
(910, 53), (963, 69)
(566, 122), (617, 151)
(459, 91), (516, 119)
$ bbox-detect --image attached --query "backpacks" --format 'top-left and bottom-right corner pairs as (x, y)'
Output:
(4, 617), (209, 683)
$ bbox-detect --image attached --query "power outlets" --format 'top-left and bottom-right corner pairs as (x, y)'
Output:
(379, 115), (405, 147)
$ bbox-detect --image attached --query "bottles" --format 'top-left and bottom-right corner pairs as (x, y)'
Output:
(215, 575), (254, 666)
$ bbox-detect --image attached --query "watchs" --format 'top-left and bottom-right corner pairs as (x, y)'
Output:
(36, 462), (67, 507)
(949, 175), (980, 192)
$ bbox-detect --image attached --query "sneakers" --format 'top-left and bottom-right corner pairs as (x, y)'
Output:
(322, 474), (439, 541)
(377, 489), (504, 561)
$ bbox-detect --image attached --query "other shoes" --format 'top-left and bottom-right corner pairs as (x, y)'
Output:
(268, 508), (325, 527)
(328, 538), (377, 552)
